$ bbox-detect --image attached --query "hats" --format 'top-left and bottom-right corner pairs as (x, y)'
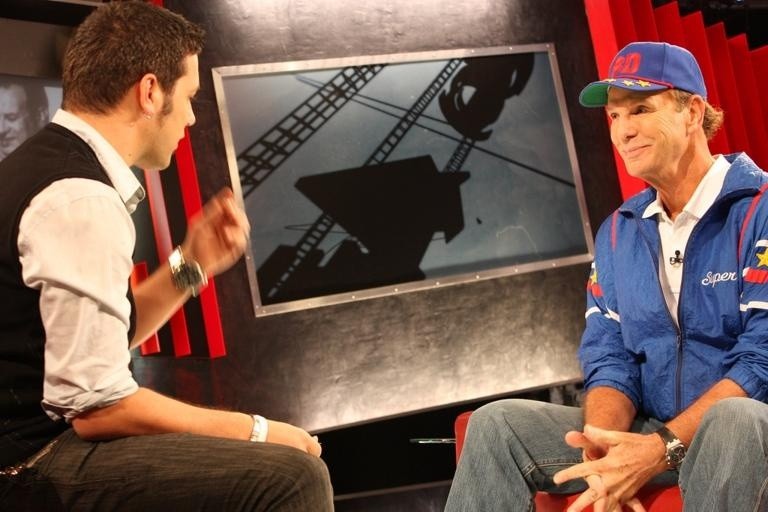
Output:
(577, 39), (707, 107)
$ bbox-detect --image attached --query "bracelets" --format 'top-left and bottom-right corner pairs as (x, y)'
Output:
(248, 413), (268, 444)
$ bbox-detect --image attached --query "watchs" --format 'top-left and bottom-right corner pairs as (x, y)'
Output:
(655, 425), (687, 474)
(165, 244), (207, 299)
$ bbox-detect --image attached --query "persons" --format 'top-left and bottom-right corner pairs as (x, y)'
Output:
(0, 1), (336, 512)
(442, 40), (768, 511)
(0, 74), (50, 158)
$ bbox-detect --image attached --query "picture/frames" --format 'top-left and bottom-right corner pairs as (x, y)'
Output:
(209, 39), (597, 322)
